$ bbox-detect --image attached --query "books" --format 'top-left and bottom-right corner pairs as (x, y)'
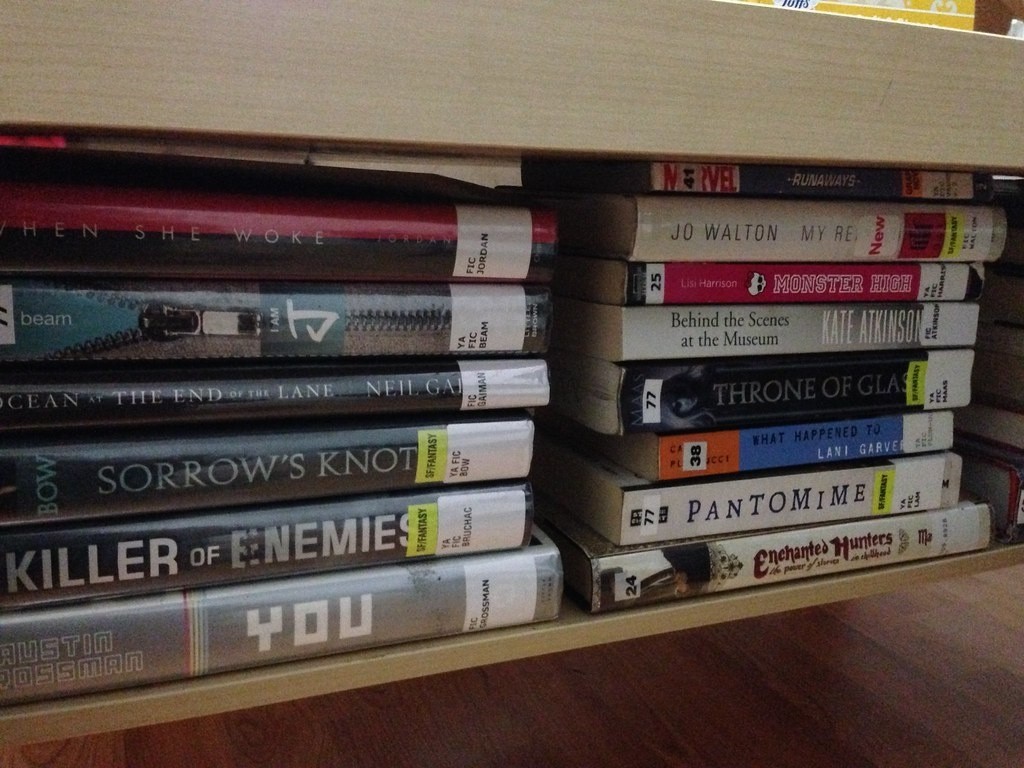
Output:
(568, 154), (1024, 611)
(0, 130), (564, 704)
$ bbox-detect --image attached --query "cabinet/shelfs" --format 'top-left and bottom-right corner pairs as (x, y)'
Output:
(0, 0), (1024, 750)
